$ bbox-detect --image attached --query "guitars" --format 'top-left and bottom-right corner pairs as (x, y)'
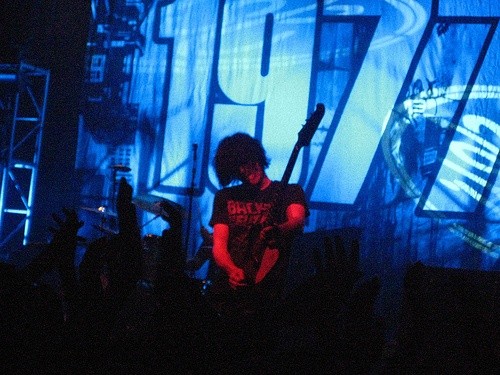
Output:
(227, 102), (325, 295)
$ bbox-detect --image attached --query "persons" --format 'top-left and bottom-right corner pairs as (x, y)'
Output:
(4, 185), (499, 312)
(205, 132), (312, 316)
